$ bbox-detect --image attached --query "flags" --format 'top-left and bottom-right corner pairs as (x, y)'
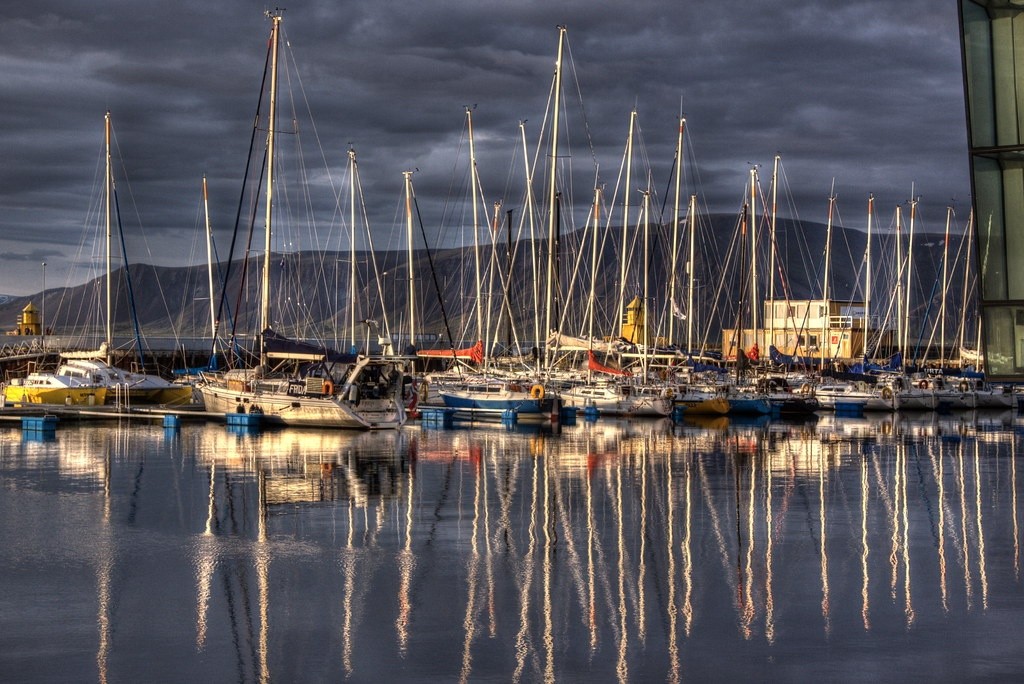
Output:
(407, 378), (418, 410)
(671, 296), (686, 320)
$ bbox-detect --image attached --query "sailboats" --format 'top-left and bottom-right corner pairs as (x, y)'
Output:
(1, 3), (1024, 432)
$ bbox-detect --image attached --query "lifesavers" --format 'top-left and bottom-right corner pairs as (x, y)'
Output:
(531, 385), (545, 400)
(919, 379), (929, 390)
(959, 382), (970, 392)
(881, 386), (892, 398)
(801, 382), (810, 395)
(320, 381), (333, 395)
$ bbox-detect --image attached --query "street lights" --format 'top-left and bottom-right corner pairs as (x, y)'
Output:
(381, 271), (389, 338)
(41, 262), (49, 337)
